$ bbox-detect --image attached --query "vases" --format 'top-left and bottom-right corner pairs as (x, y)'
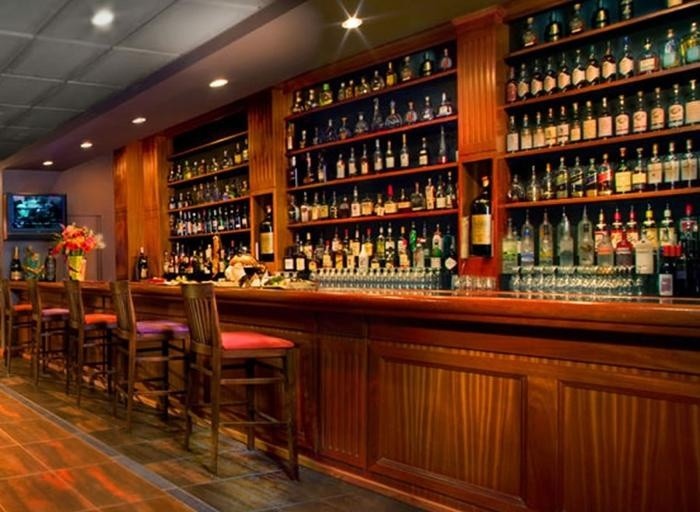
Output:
(64, 255), (87, 281)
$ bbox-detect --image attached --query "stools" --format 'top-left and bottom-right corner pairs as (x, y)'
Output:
(2, 278), (49, 375)
(63, 278), (118, 407)
(107, 279), (193, 434)
(179, 281), (297, 475)
(26, 277), (72, 387)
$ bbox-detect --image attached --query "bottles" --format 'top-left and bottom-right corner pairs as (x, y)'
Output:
(162, 138), (250, 281)
(499, 1), (700, 299)
(283, 46), (458, 291)
(43, 245), (57, 282)
(469, 176), (492, 257)
(257, 205), (274, 262)
(137, 247), (149, 281)
(10, 243), (23, 281)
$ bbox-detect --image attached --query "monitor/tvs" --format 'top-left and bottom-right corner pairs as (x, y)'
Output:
(4, 192), (67, 240)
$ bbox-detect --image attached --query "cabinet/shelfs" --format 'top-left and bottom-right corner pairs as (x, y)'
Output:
(161, 1), (700, 309)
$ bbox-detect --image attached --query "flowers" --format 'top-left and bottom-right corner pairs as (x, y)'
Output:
(49, 221), (107, 257)
